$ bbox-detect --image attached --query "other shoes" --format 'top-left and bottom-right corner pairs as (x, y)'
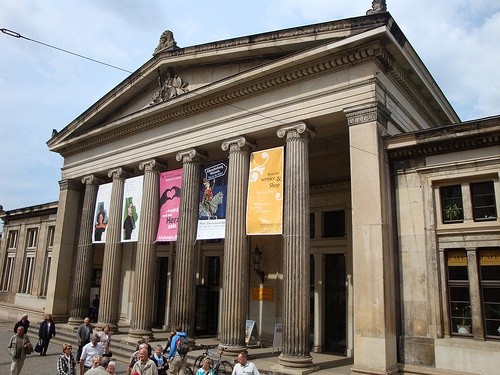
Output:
(40, 353), (47, 356)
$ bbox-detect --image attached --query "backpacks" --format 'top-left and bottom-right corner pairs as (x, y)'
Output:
(175, 336), (190, 355)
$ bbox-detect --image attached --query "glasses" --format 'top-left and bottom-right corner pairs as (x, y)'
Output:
(67, 347), (73, 350)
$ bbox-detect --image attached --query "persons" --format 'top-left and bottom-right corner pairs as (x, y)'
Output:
(83, 326), (260, 375)
(91, 294), (100, 323)
(124, 202), (134, 240)
(38, 314), (56, 356)
(203, 179), (217, 220)
(55, 343), (77, 375)
(8, 326), (34, 375)
(94, 212), (108, 243)
(14, 314), (30, 335)
(79, 334), (104, 375)
(98, 325), (111, 359)
(76, 317), (94, 363)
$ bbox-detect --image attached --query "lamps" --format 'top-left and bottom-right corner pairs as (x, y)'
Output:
(253, 244), (264, 283)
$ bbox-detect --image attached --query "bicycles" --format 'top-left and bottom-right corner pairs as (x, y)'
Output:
(193, 342), (233, 375)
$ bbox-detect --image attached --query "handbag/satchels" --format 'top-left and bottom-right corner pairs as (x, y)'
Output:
(34, 339), (43, 353)
(24, 342), (32, 355)
(108, 352), (112, 357)
